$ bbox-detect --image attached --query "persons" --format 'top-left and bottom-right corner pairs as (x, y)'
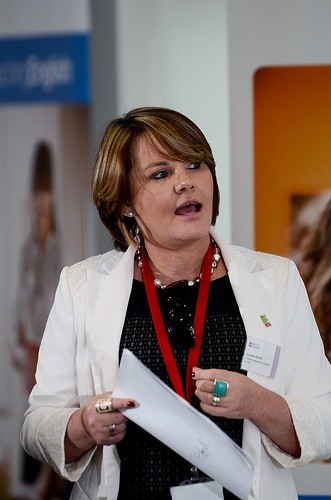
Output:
(18, 108), (331, 500)
(14, 140), (72, 500)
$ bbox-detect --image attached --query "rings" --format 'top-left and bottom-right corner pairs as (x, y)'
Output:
(212, 397), (220, 406)
(108, 424), (118, 437)
(96, 398), (112, 414)
(211, 379), (230, 397)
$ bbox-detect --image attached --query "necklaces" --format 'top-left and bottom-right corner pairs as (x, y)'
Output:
(135, 238), (221, 351)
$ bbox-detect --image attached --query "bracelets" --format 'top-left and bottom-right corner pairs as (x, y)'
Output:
(79, 406), (92, 439)
